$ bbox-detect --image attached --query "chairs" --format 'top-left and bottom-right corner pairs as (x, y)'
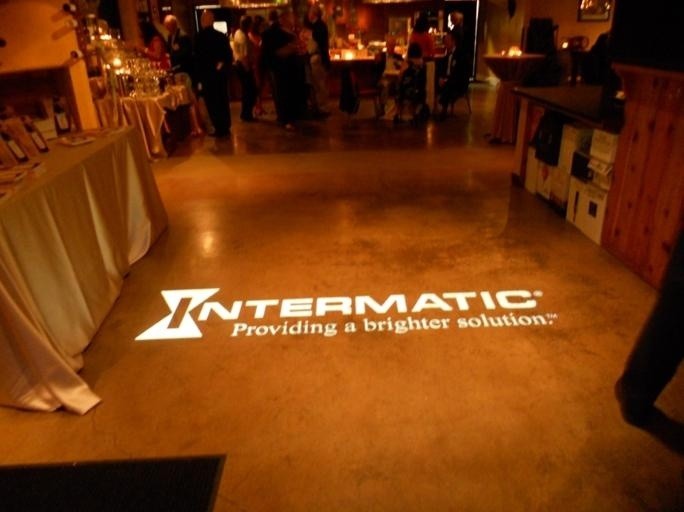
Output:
(342, 64), (383, 127)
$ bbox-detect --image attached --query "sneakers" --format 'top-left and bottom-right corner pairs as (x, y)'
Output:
(208, 112), (448, 138)
(616, 375), (655, 428)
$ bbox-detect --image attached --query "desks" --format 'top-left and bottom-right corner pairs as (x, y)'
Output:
(0, 126), (167, 411)
(482, 53), (546, 145)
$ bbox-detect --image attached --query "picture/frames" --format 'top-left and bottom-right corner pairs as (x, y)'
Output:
(576, 1), (612, 22)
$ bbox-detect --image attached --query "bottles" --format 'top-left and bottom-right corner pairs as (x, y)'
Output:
(0, 131), (28, 164)
(52, 97), (70, 134)
(20, 117), (48, 154)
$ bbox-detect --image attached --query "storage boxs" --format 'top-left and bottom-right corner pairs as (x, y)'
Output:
(524, 121), (619, 247)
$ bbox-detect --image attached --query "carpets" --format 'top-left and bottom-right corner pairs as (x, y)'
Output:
(0, 452), (227, 512)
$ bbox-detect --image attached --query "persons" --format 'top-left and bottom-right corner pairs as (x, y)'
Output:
(613, 202), (682, 457)
